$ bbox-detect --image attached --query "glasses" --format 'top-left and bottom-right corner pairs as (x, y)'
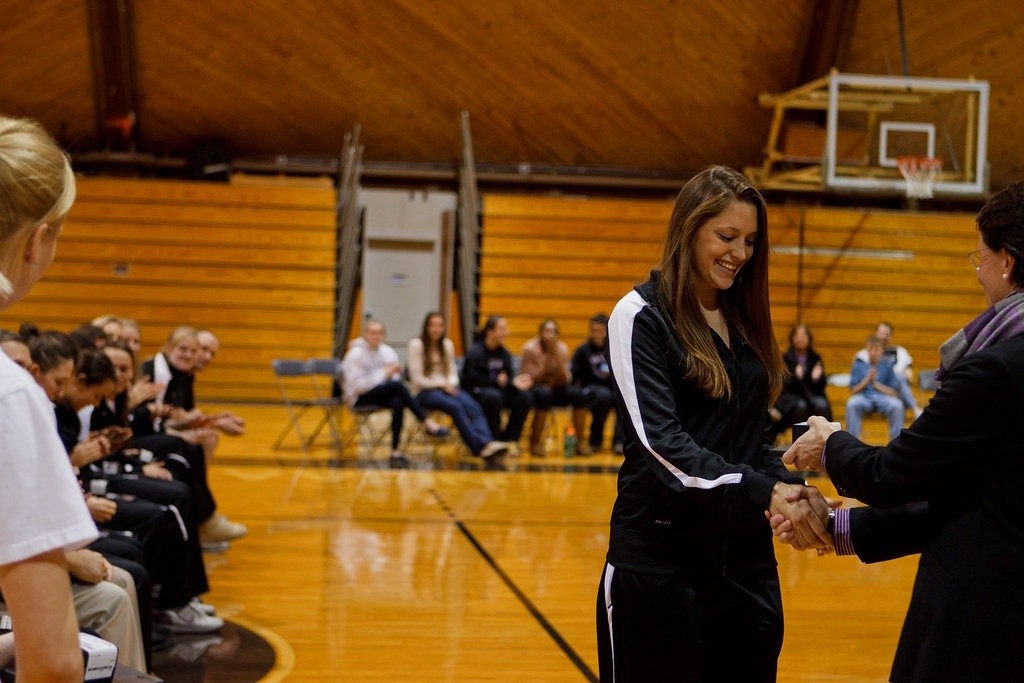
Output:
(968, 247), (991, 268)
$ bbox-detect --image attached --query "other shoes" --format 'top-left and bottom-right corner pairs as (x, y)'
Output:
(480, 441), (508, 462)
(530, 440), (544, 455)
(199, 541), (231, 553)
(613, 441), (623, 454)
(426, 426), (448, 437)
(577, 438), (592, 454)
(199, 513), (247, 543)
(151, 598), (225, 632)
(508, 441), (520, 457)
(390, 456), (409, 469)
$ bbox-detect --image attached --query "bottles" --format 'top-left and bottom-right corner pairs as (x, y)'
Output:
(565, 427), (577, 457)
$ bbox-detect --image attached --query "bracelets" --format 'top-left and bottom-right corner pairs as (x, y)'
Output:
(827, 508), (834, 535)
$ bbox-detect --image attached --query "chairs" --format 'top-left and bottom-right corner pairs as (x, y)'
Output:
(269, 355), (577, 465)
(827, 369), (939, 430)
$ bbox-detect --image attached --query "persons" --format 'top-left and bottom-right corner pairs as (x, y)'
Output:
(845, 335), (904, 446)
(769, 175), (1024, 683)
(458, 315), (530, 458)
(594, 164), (842, 683)
(569, 312), (623, 454)
(343, 317), (450, 461)
(873, 320), (924, 420)
(768, 322), (833, 444)
(54, 347), (148, 658)
(18, 318), (154, 675)
(72, 314), (247, 633)
(408, 312), (509, 460)
(0, 329), (148, 674)
(520, 317), (591, 458)
(0, 114), (100, 683)
